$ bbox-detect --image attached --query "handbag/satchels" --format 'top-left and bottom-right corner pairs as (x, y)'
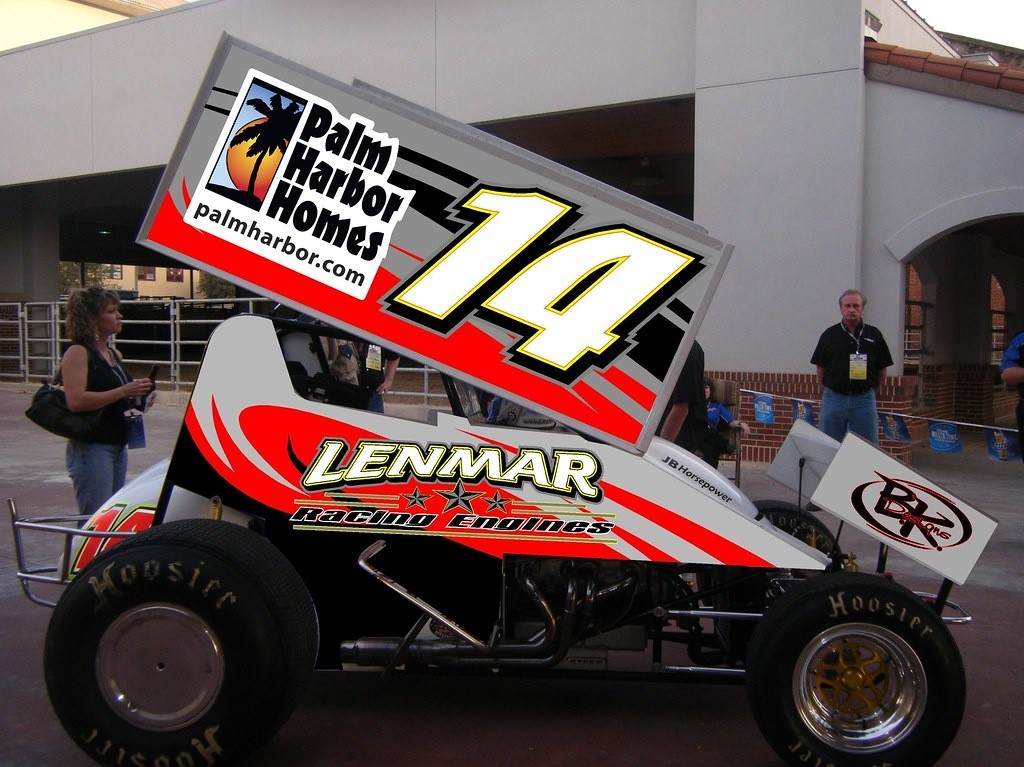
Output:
(24, 342), (107, 445)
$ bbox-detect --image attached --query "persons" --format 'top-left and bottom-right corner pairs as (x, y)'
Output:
(653, 339), (708, 459)
(704, 375), (750, 470)
(359, 341), (400, 413)
(62, 286), (157, 528)
(807, 289), (894, 511)
(999, 332), (1024, 462)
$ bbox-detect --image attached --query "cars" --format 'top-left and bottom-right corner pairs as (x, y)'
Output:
(4, 28), (999, 767)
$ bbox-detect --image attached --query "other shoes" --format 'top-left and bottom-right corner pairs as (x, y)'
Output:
(726, 443), (736, 455)
(805, 502), (821, 512)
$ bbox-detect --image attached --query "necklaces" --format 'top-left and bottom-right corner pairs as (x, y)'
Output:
(104, 350), (128, 385)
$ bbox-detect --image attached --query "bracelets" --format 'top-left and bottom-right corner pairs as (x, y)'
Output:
(739, 421), (747, 425)
